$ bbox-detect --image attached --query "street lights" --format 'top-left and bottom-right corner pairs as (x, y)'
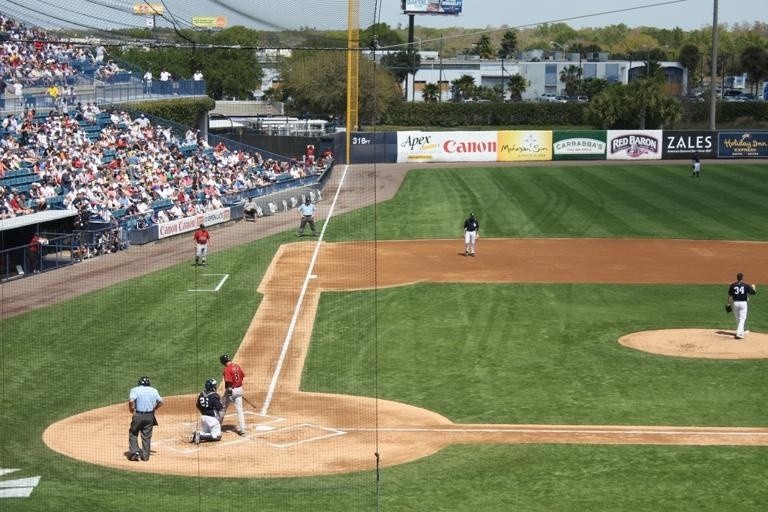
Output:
(550, 41), (565, 60)
(642, 44), (669, 79)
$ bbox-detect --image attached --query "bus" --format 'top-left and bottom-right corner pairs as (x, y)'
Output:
(725, 76), (746, 89)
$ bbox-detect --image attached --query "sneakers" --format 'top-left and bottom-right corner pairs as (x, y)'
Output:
(240, 430), (244, 435)
(130, 452), (139, 461)
(189, 432), (200, 445)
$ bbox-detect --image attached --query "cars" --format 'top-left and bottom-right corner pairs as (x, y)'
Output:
(459, 97), (492, 103)
(538, 92), (589, 104)
(462, 100), (493, 103)
(714, 89), (755, 101)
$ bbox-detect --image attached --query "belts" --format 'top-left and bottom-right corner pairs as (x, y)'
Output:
(136, 411), (153, 414)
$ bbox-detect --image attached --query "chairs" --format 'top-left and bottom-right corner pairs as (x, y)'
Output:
(0, 106), (302, 232)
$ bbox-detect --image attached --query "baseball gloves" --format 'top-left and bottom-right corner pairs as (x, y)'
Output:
(726, 304), (732, 312)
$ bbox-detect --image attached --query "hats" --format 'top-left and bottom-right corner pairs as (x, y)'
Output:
(200, 223), (205, 229)
(471, 213), (475, 217)
(307, 197), (311, 203)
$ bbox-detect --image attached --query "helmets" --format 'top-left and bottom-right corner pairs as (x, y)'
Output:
(204, 379), (217, 391)
(220, 355), (231, 364)
(139, 376), (150, 386)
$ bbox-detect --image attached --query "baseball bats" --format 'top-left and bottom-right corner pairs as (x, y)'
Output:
(242, 397), (256, 409)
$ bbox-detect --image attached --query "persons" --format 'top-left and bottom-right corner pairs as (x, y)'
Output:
(218, 354), (247, 437)
(463, 212), (480, 256)
(725, 272), (758, 340)
(692, 150), (701, 177)
(0, 11), (334, 273)
(188, 377), (223, 445)
(126, 375), (165, 461)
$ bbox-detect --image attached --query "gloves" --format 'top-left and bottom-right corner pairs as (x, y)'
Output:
(228, 394), (233, 402)
